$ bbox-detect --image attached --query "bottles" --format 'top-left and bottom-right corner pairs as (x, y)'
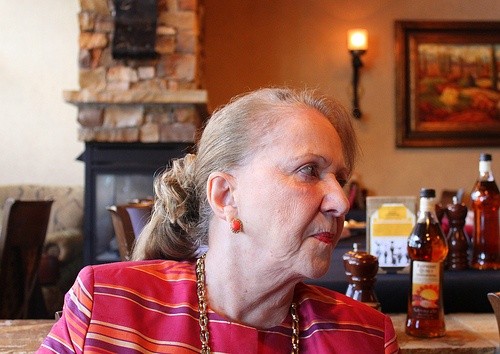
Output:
(470, 152), (500, 271)
(446, 196), (471, 272)
(405, 188), (448, 337)
(341, 250), (380, 311)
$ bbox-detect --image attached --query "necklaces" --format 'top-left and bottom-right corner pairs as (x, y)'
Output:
(195, 257), (300, 354)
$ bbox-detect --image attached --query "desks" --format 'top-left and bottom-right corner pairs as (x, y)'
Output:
(306, 249), (500, 314)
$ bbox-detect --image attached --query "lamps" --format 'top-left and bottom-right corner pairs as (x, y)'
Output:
(347, 28), (369, 118)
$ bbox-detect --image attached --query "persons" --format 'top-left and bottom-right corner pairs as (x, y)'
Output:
(33, 86), (402, 354)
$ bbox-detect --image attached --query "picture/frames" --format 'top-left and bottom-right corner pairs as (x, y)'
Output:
(393, 20), (500, 150)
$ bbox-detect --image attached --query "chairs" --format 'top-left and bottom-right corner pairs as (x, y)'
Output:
(105, 201), (154, 261)
(0, 197), (56, 319)
